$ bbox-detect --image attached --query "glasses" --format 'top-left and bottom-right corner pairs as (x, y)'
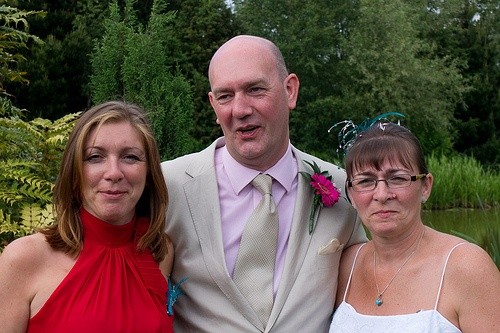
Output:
(348, 174), (426, 192)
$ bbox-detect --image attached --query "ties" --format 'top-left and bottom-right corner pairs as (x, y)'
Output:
(233, 174), (279, 330)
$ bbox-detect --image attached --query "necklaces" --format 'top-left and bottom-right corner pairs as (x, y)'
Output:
(373, 229), (425, 305)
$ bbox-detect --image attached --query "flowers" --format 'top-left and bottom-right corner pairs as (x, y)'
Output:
(298, 159), (340, 235)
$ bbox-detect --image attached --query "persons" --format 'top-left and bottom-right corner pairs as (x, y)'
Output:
(0, 101), (190, 333)
(158, 35), (370, 333)
(329, 113), (500, 333)
